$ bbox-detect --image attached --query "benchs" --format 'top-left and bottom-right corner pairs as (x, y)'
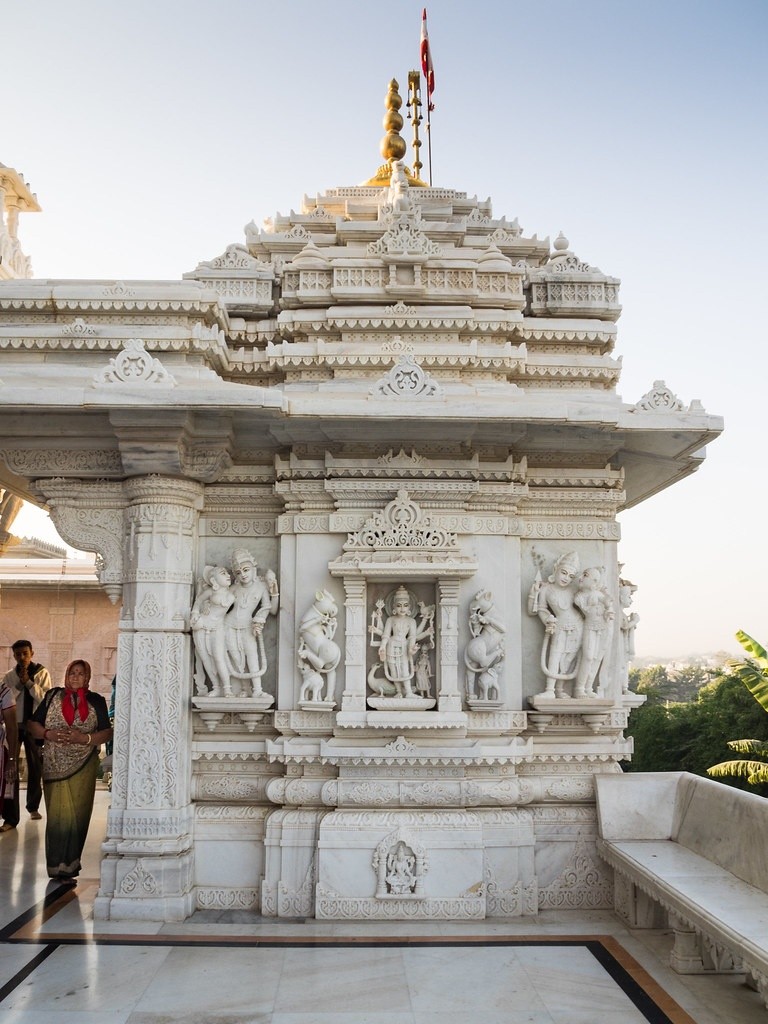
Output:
(592, 771), (768, 1008)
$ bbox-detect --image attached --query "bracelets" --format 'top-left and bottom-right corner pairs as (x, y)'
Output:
(86, 734), (91, 744)
(43, 729), (51, 740)
(10, 757), (17, 761)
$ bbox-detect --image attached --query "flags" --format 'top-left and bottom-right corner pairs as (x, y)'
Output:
(421, 6), (441, 102)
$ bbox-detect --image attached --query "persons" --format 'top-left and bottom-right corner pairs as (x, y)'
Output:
(3, 640), (53, 834)
(103, 673), (115, 788)
(0, 683), (19, 802)
(195, 548), (644, 715)
(26, 660), (113, 886)
